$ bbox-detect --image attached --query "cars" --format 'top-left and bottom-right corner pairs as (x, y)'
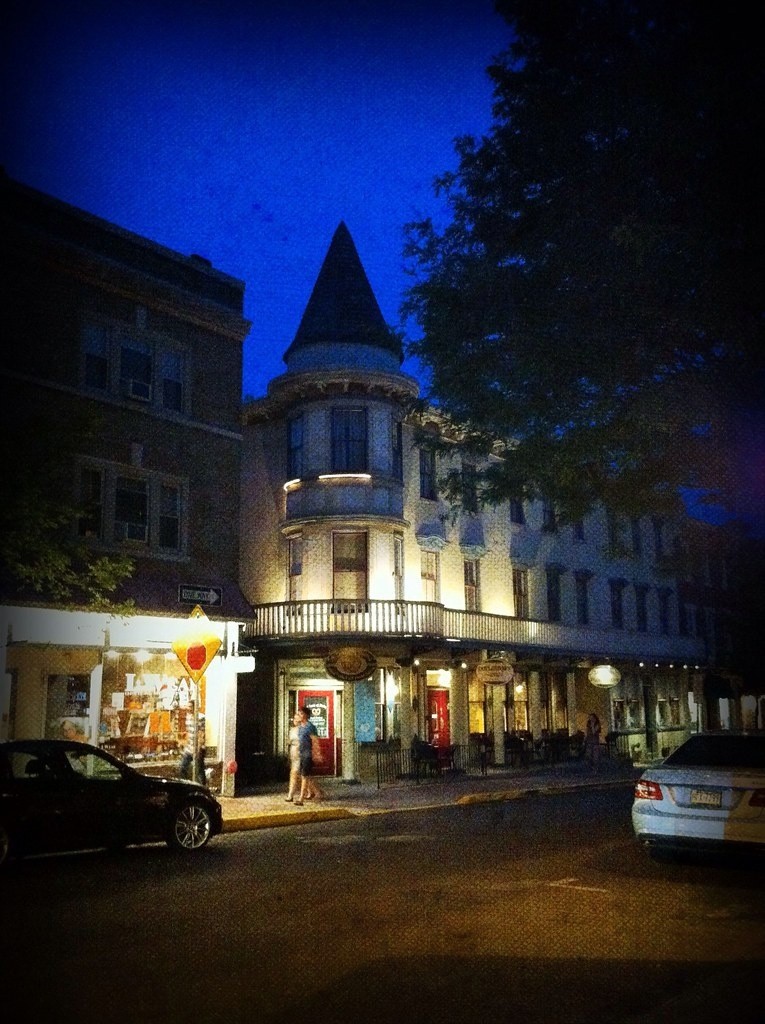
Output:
(0, 738), (225, 858)
(630, 728), (765, 864)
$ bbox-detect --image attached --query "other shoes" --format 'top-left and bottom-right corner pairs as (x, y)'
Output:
(294, 800), (303, 805)
(304, 791), (314, 799)
(285, 797), (293, 802)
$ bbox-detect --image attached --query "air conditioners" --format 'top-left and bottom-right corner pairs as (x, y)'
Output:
(122, 378), (153, 402)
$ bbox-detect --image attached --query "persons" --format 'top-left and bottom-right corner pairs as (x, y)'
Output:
(584, 714), (601, 777)
(181, 700), (207, 786)
(283, 707), (323, 807)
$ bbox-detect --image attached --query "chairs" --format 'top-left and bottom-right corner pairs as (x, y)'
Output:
(505, 728), (621, 767)
